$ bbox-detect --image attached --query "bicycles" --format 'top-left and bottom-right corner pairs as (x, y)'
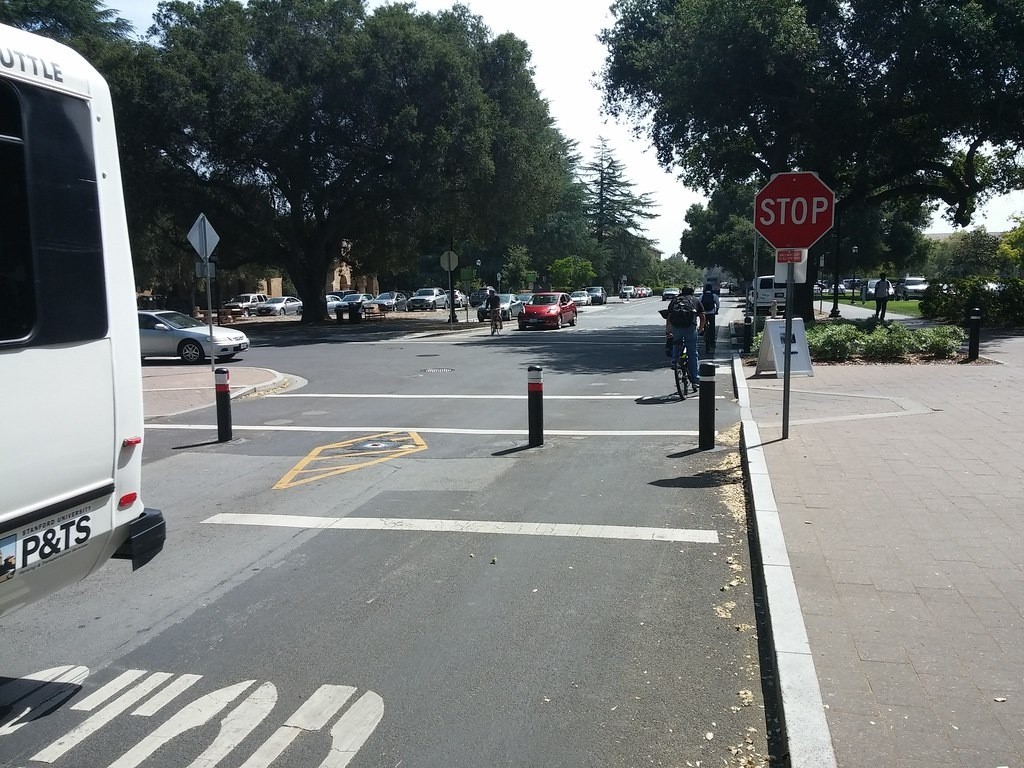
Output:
(699, 312), (718, 353)
(486, 308), (501, 336)
(666, 328), (700, 400)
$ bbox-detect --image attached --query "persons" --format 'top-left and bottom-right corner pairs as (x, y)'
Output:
(486, 289), (503, 330)
(699, 284), (720, 341)
(666, 286), (706, 386)
(875, 273), (889, 321)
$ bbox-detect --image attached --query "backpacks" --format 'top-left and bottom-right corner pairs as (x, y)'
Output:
(670, 294), (697, 327)
(701, 291), (716, 311)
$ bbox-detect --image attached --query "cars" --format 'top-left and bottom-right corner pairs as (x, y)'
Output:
(646, 288), (653, 296)
(257, 296), (303, 316)
(866, 280), (894, 301)
(571, 291), (592, 306)
(337, 294), (375, 312)
(518, 293), (578, 329)
(326, 295), (341, 313)
(636, 288), (644, 298)
(693, 289), (703, 297)
(476, 294), (524, 321)
(138, 310), (250, 366)
(828, 284), (846, 294)
(371, 292), (408, 312)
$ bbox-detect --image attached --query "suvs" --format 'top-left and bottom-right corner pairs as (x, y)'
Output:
(585, 287), (608, 305)
(445, 290), (468, 307)
(895, 277), (930, 301)
(407, 288), (450, 310)
(662, 287), (679, 301)
(224, 294), (269, 318)
(470, 286), (496, 308)
(619, 286), (637, 299)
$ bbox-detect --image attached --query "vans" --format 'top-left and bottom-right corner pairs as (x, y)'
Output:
(328, 290), (357, 299)
(749, 275), (787, 311)
(0, 27), (166, 622)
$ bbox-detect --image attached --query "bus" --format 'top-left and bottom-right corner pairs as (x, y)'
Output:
(703, 274), (720, 295)
(843, 279), (861, 289)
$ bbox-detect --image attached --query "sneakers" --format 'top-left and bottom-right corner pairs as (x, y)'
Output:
(671, 363), (683, 370)
(693, 382), (700, 388)
